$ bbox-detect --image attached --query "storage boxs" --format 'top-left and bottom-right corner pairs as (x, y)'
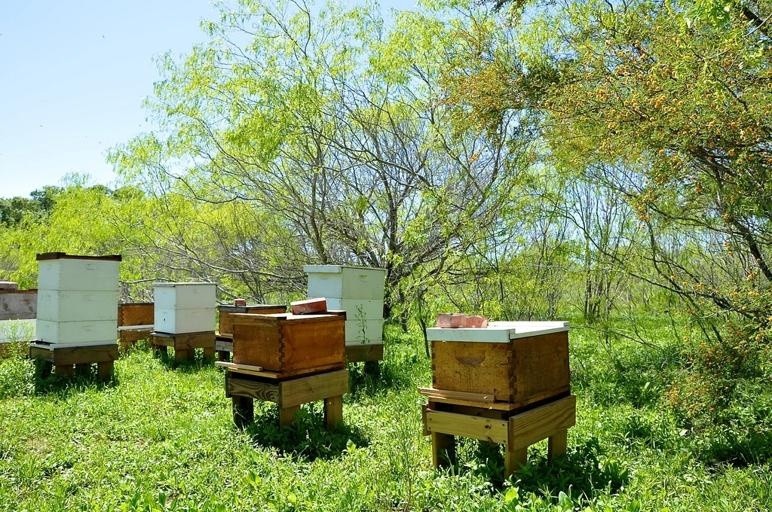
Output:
(118, 304), (155, 325)
(428, 321), (570, 404)
(218, 305), (347, 370)
